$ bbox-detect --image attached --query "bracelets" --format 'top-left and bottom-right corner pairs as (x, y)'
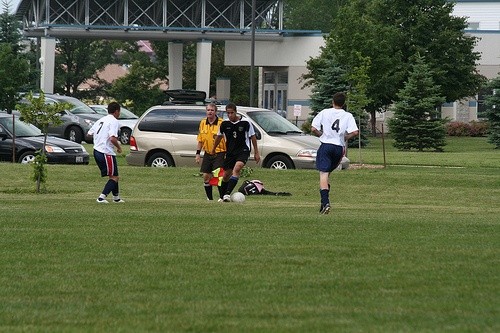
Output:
(196, 150), (200, 155)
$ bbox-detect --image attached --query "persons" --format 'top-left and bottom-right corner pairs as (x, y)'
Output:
(212, 103), (260, 202)
(86, 102), (126, 203)
(311, 93), (358, 215)
(196, 103), (227, 200)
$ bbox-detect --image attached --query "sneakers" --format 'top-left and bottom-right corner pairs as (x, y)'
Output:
(113, 199), (124, 202)
(320, 203), (331, 214)
(97, 198), (109, 203)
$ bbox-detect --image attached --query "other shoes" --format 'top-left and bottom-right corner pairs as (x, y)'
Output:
(218, 198), (223, 202)
(223, 195), (230, 202)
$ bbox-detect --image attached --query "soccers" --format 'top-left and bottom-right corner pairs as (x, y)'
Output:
(231, 192), (245, 202)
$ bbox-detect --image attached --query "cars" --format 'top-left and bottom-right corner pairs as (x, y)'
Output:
(69, 104), (140, 145)
(0, 109), (90, 165)
(18, 93), (105, 144)
(126, 88), (351, 172)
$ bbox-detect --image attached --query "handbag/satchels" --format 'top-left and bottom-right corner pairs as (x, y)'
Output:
(238, 180), (263, 196)
